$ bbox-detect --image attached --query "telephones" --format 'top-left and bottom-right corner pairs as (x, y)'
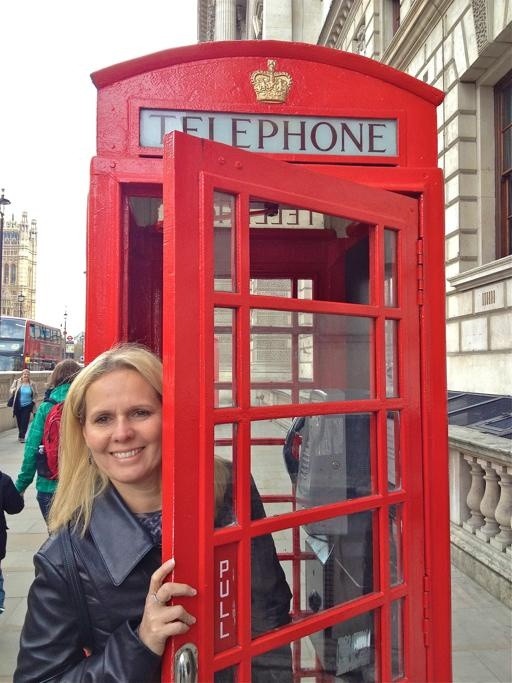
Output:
(283, 388), (373, 536)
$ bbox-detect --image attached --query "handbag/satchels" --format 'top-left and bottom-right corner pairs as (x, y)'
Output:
(6, 395), (14, 409)
(37, 398), (63, 480)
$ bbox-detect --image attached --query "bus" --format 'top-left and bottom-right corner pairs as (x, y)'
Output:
(0, 316), (64, 372)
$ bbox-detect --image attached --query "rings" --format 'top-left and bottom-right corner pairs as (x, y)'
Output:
(154, 595), (165, 605)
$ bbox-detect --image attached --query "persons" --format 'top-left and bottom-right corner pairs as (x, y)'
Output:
(15, 359), (82, 525)
(12, 343), (293, 683)
(0, 471), (24, 613)
(9, 369), (39, 443)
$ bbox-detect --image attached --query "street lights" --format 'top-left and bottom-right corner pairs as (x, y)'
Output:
(63, 310), (68, 359)
(0, 195), (11, 288)
(17, 290), (25, 317)
(79, 333), (84, 364)
